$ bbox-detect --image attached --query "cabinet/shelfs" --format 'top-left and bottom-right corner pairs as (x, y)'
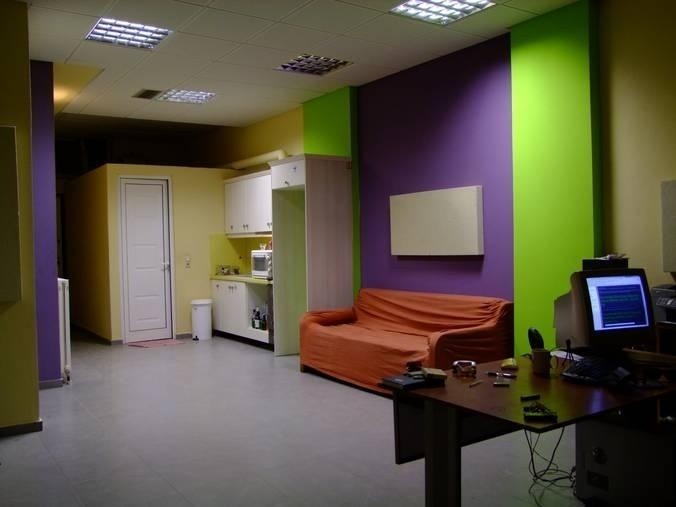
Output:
(222, 168), (274, 239)
(265, 153), (353, 357)
(208, 276), (273, 352)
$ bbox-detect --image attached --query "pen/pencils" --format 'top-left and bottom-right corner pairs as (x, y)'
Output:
(470, 379), (483, 387)
(485, 372), (517, 377)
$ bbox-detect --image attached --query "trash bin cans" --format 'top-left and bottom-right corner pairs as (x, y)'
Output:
(191, 298), (212, 341)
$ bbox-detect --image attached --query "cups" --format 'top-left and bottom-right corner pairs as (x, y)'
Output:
(532, 349), (559, 379)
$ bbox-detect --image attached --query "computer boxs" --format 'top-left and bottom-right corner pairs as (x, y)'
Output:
(576, 419), (676, 507)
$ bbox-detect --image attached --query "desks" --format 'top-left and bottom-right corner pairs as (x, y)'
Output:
(376, 347), (676, 507)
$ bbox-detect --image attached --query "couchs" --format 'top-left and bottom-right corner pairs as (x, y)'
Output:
(300, 287), (513, 396)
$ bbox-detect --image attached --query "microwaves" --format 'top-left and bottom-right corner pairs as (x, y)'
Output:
(250, 250), (273, 279)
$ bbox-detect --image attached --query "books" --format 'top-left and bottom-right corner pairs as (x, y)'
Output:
(382, 374), (444, 390)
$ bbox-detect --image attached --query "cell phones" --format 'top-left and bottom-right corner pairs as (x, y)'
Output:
(524, 411), (558, 422)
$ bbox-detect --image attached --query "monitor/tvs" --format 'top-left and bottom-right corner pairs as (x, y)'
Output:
(555, 268), (655, 384)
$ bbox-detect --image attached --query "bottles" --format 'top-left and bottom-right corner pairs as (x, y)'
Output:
(251, 304), (267, 330)
